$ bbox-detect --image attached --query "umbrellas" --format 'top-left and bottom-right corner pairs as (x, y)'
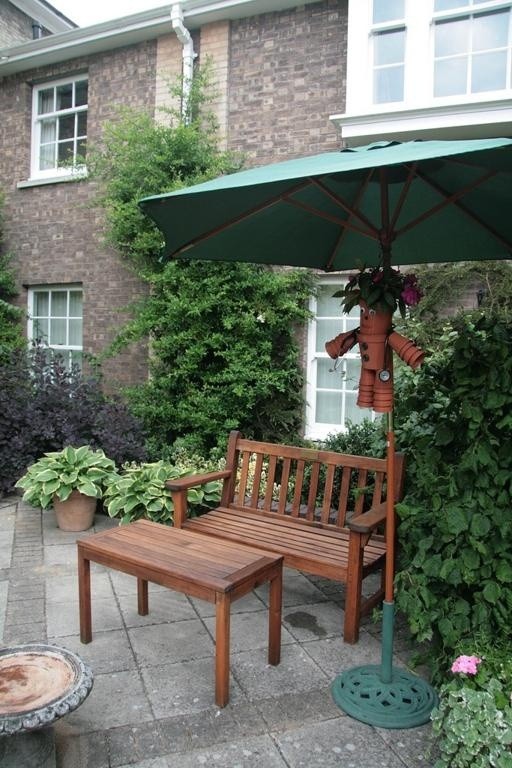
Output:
(131, 137), (509, 609)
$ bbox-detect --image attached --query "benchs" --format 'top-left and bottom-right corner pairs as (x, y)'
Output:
(165, 429), (411, 648)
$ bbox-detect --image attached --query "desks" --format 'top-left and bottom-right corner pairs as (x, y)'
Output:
(74, 515), (286, 713)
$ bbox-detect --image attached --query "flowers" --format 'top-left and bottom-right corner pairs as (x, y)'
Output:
(331, 255), (425, 319)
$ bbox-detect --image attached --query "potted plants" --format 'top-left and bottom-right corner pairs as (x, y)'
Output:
(14, 444), (121, 532)
